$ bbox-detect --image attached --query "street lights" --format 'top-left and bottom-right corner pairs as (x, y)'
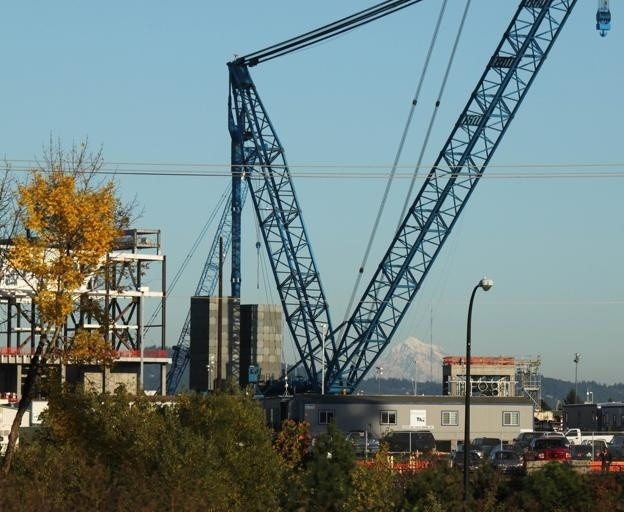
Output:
(461, 275), (494, 498)
(573, 352), (581, 403)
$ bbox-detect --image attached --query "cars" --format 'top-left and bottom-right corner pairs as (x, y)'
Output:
(448, 428), (624, 474)
(344, 431), (383, 456)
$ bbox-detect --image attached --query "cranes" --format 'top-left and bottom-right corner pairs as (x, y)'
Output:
(222, 2), (615, 398)
(137, 169), (249, 396)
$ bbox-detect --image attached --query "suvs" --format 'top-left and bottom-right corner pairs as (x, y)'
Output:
(381, 429), (438, 454)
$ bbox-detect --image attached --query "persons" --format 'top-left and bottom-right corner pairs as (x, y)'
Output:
(599, 448), (613, 473)
